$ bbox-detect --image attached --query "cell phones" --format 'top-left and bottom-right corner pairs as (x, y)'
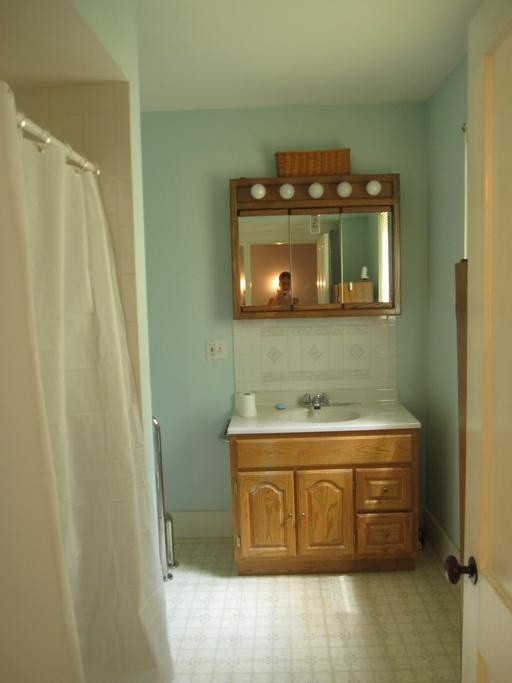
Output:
(283, 291), (289, 300)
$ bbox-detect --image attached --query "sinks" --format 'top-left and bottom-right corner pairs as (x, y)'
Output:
(279, 401), (362, 424)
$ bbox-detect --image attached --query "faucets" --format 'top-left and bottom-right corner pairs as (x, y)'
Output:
(312, 393), (323, 409)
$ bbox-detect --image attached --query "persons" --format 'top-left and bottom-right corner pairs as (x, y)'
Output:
(265, 271), (300, 307)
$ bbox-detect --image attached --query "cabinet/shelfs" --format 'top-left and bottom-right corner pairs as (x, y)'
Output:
(229, 434), (418, 577)
(330, 280), (375, 305)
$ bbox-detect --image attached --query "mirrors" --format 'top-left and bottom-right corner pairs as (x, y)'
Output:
(229, 173), (405, 322)
